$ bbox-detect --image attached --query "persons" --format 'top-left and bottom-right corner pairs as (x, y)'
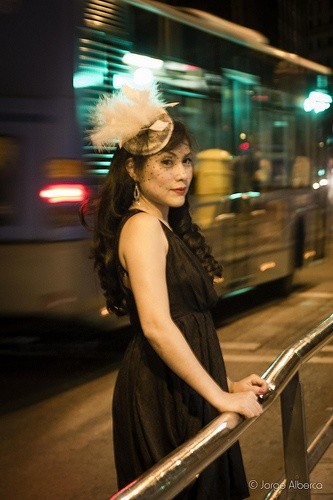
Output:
(94, 112), (269, 500)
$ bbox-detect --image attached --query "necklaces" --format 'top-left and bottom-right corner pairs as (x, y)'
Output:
(135, 200), (169, 222)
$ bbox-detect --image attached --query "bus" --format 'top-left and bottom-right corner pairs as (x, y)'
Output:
(0, 0), (331, 330)
